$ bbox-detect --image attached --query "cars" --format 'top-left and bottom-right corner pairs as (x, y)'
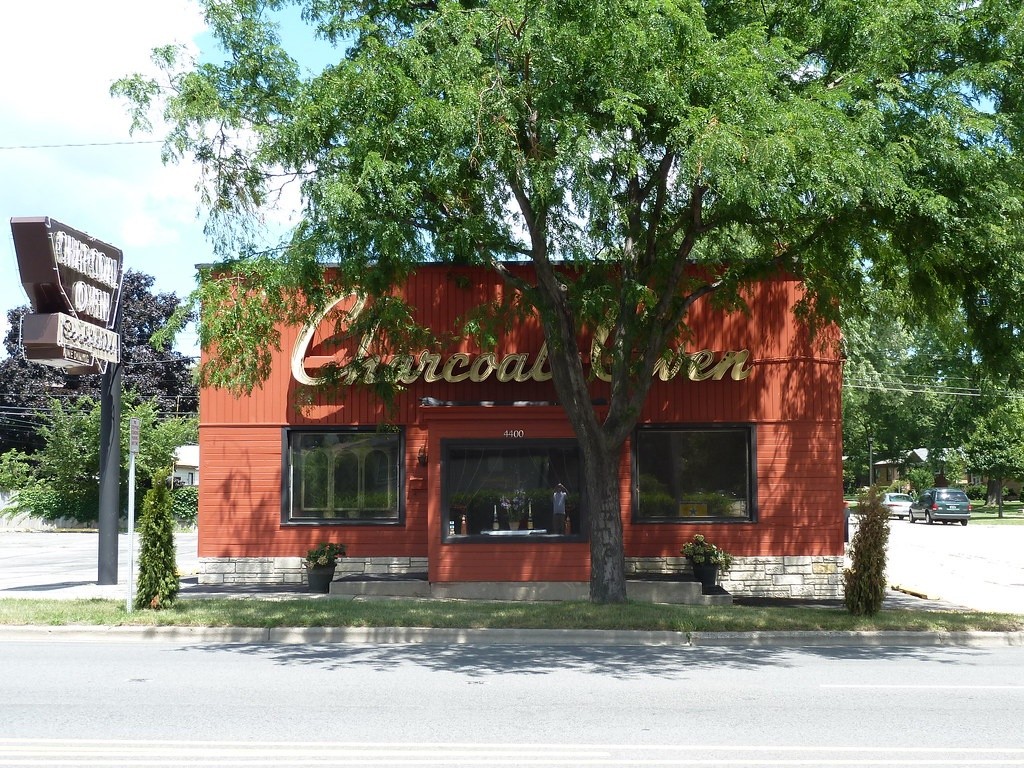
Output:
(878, 492), (915, 520)
(909, 486), (972, 526)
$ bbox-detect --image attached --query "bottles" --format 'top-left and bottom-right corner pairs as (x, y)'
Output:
(492, 514), (499, 531)
(461, 515), (467, 535)
(527, 515), (533, 530)
(565, 514), (572, 534)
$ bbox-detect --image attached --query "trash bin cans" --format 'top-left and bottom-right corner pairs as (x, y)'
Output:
(843, 501), (851, 543)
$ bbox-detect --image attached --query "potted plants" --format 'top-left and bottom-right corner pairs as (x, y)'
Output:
(304, 542), (348, 593)
(675, 492), (732, 516)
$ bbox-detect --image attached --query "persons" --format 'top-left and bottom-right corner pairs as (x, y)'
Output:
(552, 483), (569, 534)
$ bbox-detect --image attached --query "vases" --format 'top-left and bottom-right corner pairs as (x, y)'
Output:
(508, 523), (521, 530)
(691, 563), (718, 591)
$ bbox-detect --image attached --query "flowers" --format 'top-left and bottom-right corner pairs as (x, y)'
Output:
(500, 490), (529, 523)
(680, 534), (735, 574)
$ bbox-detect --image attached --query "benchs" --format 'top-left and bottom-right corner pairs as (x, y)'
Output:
(480, 529), (548, 535)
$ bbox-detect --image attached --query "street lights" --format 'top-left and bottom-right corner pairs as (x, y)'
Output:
(867, 432), (875, 487)
